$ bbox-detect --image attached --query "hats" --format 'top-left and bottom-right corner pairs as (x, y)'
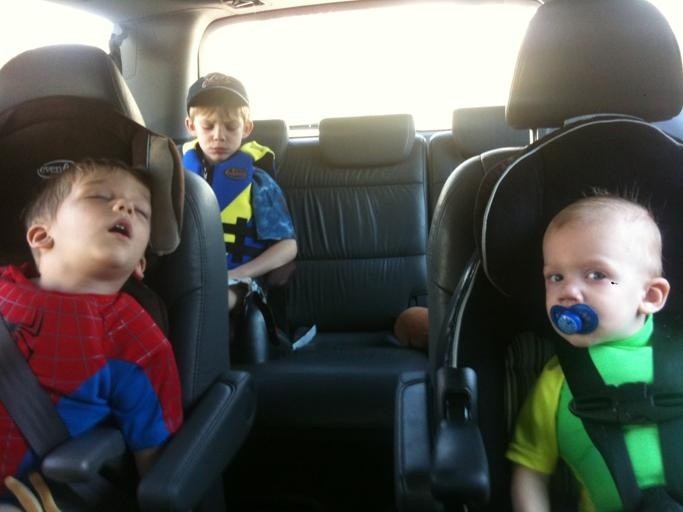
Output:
(186, 72), (248, 113)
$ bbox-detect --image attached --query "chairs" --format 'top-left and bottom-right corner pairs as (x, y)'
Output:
(393, 0), (682, 511)
(3, 43), (263, 512)
(431, 105), (537, 234)
(255, 114), (432, 431)
(173, 118), (287, 377)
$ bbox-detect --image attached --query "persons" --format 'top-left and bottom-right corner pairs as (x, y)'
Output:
(393, 306), (428, 352)
(0, 158), (183, 512)
(506, 192), (683, 512)
(175, 73), (299, 313)
